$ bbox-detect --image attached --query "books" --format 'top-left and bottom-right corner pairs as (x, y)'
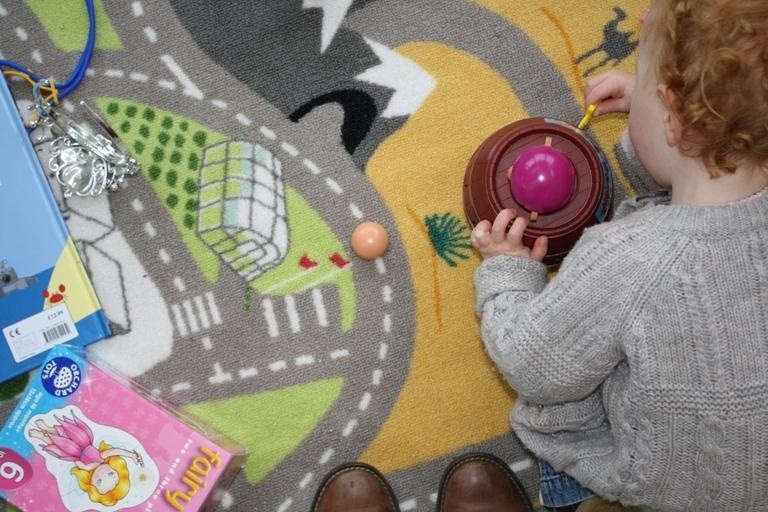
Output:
(1, 70), (114, 389)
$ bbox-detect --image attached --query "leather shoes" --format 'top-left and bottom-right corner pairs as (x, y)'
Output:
(436, 452), (534, 512)
(309, 461), (401, 512)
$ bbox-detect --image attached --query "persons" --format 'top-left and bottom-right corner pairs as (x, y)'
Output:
(466, 1), (765, 511)
